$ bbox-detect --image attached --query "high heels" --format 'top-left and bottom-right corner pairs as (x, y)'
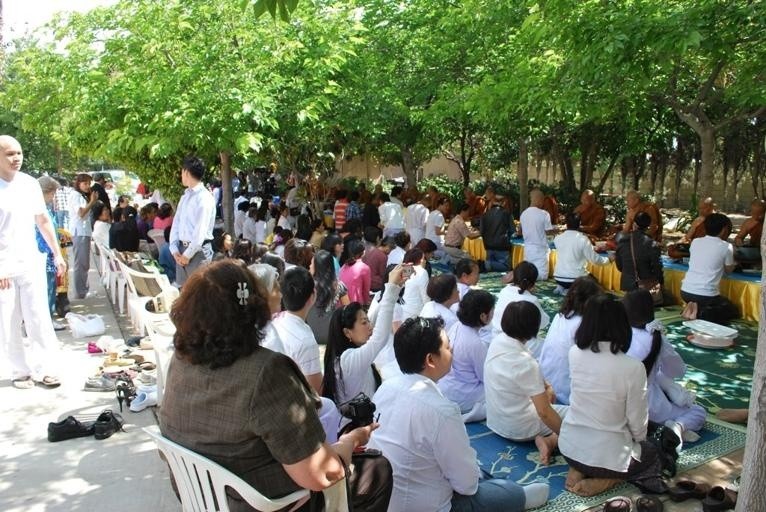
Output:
(701, 484), (739, 512)
(667, 478), (715, 503)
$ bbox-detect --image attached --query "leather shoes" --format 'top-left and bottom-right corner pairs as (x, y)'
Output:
(90, 408), (128, 442)
(46, 413), (95, 444)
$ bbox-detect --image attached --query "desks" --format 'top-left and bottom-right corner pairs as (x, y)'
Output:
(461, 228), (762, 324)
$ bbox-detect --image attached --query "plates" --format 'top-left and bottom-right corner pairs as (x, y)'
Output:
(686, 334), (735, 351)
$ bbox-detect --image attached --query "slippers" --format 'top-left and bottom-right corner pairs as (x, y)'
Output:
(634, 494), (665, 511)
(602, 494), (634, 511)
(10, 375), (34, 390)
(32, 373), (63, 387)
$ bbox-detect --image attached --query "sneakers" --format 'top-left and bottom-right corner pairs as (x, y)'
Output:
(83, 334), (158, 413)
(83, 289), (98, 301)
(51, 319), (66, 331)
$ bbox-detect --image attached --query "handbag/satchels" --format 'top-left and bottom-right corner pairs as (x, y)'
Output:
(636, 277), (665, 308)
(64, 311), (107, 339)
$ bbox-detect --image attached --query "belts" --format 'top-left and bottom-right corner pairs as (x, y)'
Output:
(177, 238), (214, 249)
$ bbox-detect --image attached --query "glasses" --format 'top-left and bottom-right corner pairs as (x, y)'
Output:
(413, 314), (431, 342)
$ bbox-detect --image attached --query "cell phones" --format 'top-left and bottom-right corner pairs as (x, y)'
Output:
(352, 448), (382, 459)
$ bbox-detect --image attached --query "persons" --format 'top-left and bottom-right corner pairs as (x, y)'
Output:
(0, 134), (68, 389)
(34, 155), (766, 511)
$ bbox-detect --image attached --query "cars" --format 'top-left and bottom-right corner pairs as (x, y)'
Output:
(26, 170), (145, 216)
(217, 165), (295, 224)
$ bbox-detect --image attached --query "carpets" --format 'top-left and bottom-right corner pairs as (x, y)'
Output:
(425, 247), (515, 283)
(460, 408), (748, 511)
(457, 271), (762, 423)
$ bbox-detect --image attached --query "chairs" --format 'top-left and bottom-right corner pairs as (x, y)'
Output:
(87, 231), (181, 412)
(138, 426), (354, 512)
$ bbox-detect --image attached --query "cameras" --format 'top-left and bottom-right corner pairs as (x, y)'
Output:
(402, 266), (415, 277)
(340, 391), (378, 429)
